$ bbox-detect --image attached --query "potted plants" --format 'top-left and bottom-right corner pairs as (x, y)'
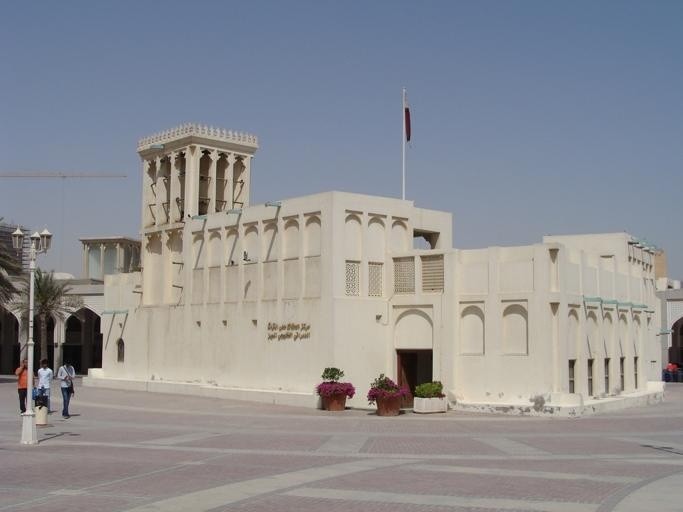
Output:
(413, 381), (449, 414)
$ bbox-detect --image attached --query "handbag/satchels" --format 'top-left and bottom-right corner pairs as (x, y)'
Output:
(32, 388), (48, 407)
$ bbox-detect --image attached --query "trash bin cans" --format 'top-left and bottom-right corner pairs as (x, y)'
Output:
(663, 368), (683, 382)
(34, 395), (48, 425)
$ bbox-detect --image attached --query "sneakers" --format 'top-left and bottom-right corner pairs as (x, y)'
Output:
(64, 415), (70, 418)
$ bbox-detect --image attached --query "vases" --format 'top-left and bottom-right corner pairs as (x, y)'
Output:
(322, 394), (346, 411)
(375, 397), (402, 417)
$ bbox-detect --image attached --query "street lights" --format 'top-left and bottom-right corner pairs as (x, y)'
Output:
(12, 225), (52, 444)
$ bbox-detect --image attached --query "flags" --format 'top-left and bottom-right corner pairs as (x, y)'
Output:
(405, 90), (411, 141)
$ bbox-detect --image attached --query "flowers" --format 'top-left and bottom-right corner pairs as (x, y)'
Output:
(367, 373), (413, 406)
(317, 381), (356, 399)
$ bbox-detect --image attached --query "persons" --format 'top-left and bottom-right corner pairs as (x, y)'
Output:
(666, 361), (674, 382)
(15, 359), (35, 414)
(37, 359), (53, 413)
(56, 360), (76, 419)
